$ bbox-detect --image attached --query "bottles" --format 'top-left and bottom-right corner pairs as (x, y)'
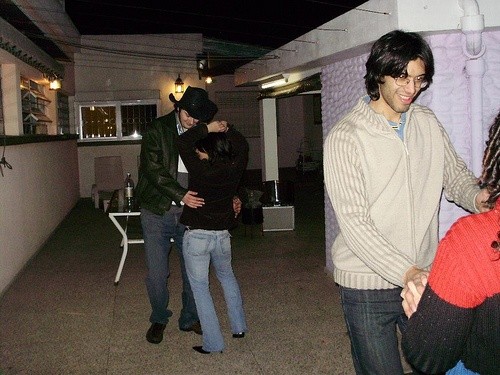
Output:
(125, 173), (134, 212)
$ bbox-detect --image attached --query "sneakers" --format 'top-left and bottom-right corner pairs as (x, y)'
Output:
(180, 320), (203, 335)
(146, 322), (166, 344)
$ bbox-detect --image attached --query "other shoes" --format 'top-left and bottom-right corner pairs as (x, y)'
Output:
(192, 346), (224, 354)
(233, 331), (245, 338)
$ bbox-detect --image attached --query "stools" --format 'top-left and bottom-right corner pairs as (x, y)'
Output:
(242, 215), (264, 238)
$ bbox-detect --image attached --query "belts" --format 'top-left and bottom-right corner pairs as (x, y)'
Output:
(172, 201), (184, 206)
(185, 225), (228, 231)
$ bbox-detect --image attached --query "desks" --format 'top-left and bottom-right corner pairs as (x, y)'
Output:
(107, 189), (174, 287)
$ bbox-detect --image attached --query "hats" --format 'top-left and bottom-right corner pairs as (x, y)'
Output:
(169, 86), (218, 123)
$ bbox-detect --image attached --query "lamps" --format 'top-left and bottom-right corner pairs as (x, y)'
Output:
(174, 74), (184, 93)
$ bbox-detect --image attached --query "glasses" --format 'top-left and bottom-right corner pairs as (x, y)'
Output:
(392, 75), (428, 89)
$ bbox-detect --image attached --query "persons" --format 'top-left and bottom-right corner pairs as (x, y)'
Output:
(322, 30), (491, 375)
(401, 109), (500, 375)
(134, 87), (242, 343)
(176, 119), (250, 354)
